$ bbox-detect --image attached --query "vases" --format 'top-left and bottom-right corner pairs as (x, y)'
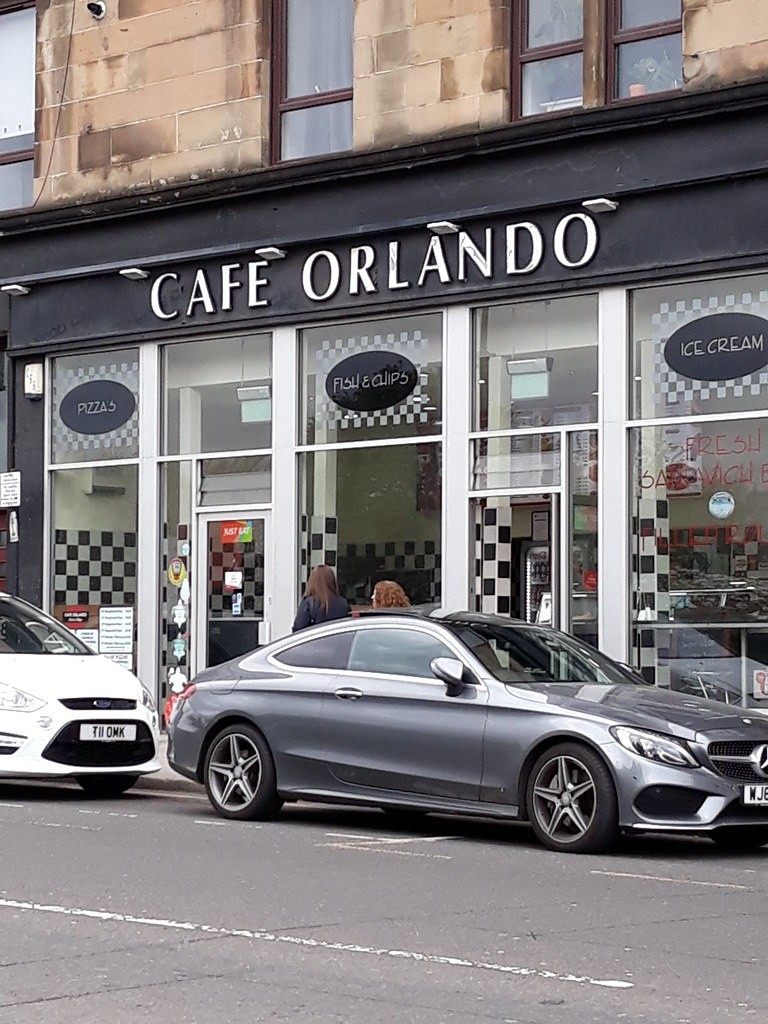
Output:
(629, 83), (646, 97)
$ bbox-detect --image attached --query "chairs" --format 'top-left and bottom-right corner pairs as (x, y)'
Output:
(366, 645), (395, 674)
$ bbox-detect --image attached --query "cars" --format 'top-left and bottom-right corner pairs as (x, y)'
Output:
(0, 591), (161, 794)
(560, 592), (768, 710)
(166, 608), (768, 858)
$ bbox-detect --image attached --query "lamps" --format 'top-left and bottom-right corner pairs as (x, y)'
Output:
(506, 300), (553, 399)
(119, 268), (150, 280)
(237, 333), (272, 422)
(1, 285), (31, 296)
(427, 221), (460, 235)
(255, 247), (287, 261)
(582, 198), (619, 213)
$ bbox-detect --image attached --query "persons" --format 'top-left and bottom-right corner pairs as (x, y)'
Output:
(370, 580), (411, 609)
(290, 565), (350, 633)
